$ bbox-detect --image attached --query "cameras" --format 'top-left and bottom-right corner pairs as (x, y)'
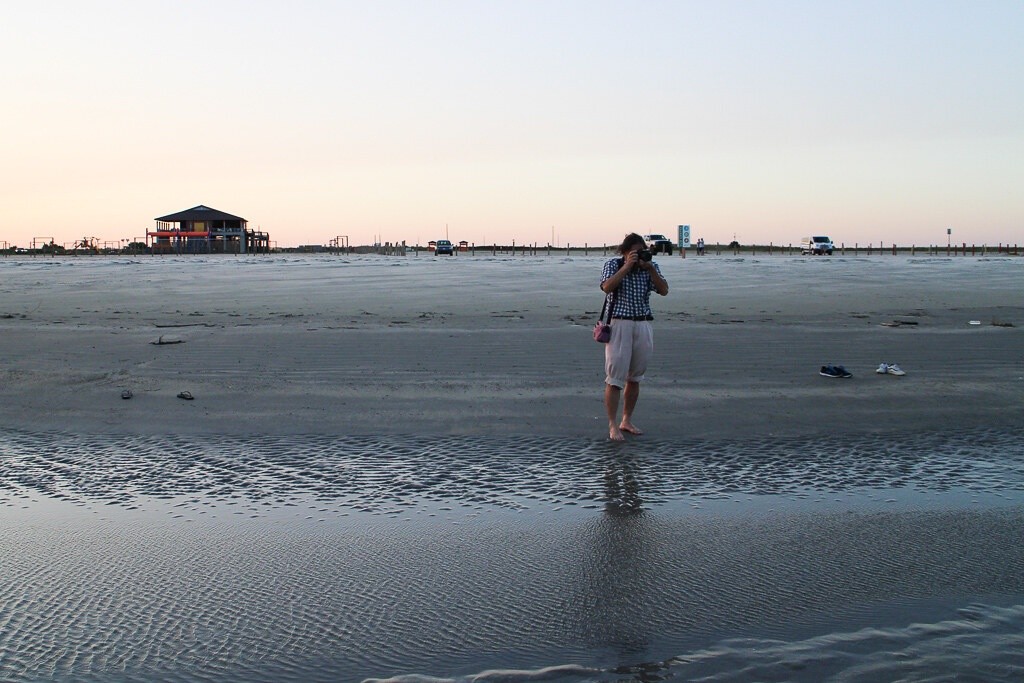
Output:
(636, 248), (652, 263)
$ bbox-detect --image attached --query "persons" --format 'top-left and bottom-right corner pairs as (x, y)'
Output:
(600, 232), (669, 441)
(697, 238), (704, 255)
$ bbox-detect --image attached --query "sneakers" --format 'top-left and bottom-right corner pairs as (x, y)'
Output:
(820, 366), (842, 377)
(887, 364), (905, 375)
(876, 363), (887, 373)
(833, 366), (853, 378)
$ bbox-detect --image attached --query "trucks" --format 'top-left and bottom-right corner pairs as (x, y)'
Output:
(800, 234), (833, 256)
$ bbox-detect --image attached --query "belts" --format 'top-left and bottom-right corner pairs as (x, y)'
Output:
(612, 315), (654, 321)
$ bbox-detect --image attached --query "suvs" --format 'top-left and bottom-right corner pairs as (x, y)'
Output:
(643, 234), (673, 256)
(433, 239), (454, 256)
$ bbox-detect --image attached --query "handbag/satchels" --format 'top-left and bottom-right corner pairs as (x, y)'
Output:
(593, 321), (610, 343)
